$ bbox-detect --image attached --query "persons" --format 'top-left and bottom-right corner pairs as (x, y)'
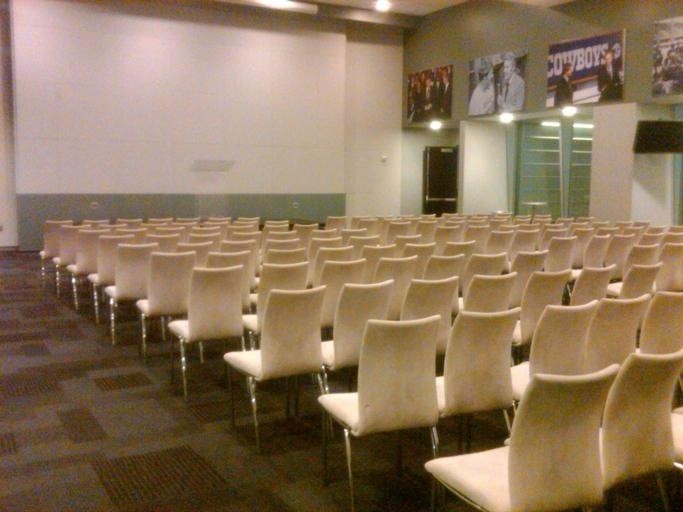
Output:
(597, 48), (621, 91)
(653, 38), (683, 94)
(468, 61), (495, 115)
(407, 65), (452, 122)
(554, 62), (573, 106)
(496, 55), (525, 113)
